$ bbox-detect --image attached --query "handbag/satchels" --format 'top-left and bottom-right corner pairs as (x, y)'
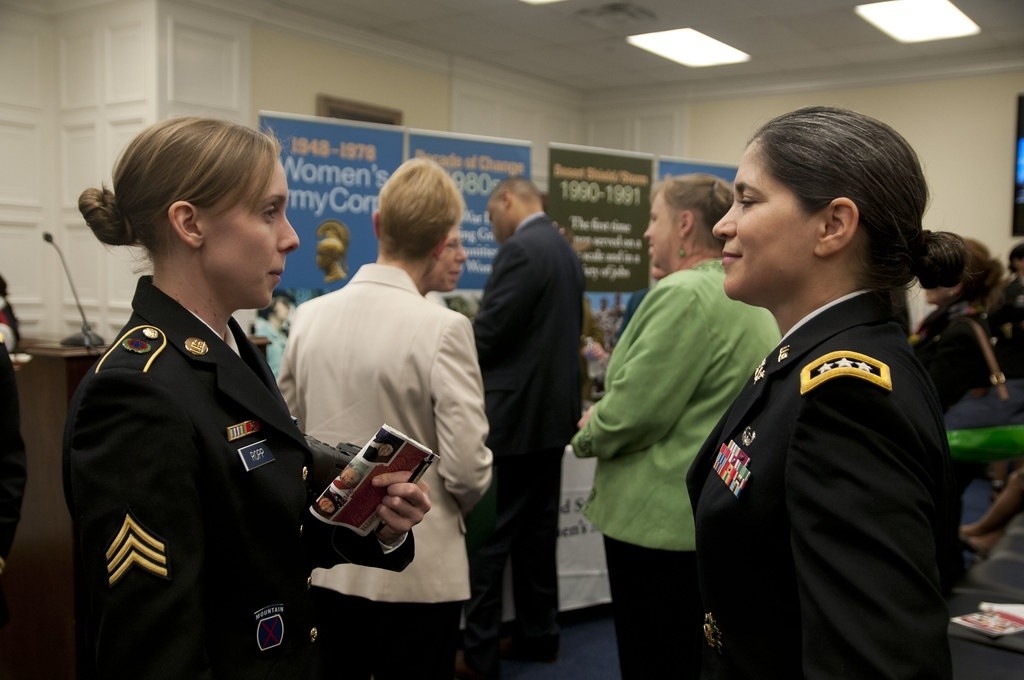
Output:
(943, 378), (1024, 430)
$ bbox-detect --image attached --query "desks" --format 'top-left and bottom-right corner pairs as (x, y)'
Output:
(25, 334), (272, 410)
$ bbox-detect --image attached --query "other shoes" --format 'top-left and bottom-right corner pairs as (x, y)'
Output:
(454, 650), (501, 680)
(957, 523), (991, 560)
(500, 636), (560, 662)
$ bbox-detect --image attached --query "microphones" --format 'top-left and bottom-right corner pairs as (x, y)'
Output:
(43, 233), (105, 347)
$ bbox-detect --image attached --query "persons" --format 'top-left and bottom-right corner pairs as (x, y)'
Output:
(311, 487), (339, 517)
(683, 106), (969, 680)
(455, 177), (585, 680)
(366, 436), (395, 460)
(906, 237), (1024, 558)
(56, 116), (433, 680)
(0, 342), (28, 618)
(569, 170), (782, 680)
(274, 155), (494, 680)
(581, 260), (670, 403)
(339, 463), (365, 489)
